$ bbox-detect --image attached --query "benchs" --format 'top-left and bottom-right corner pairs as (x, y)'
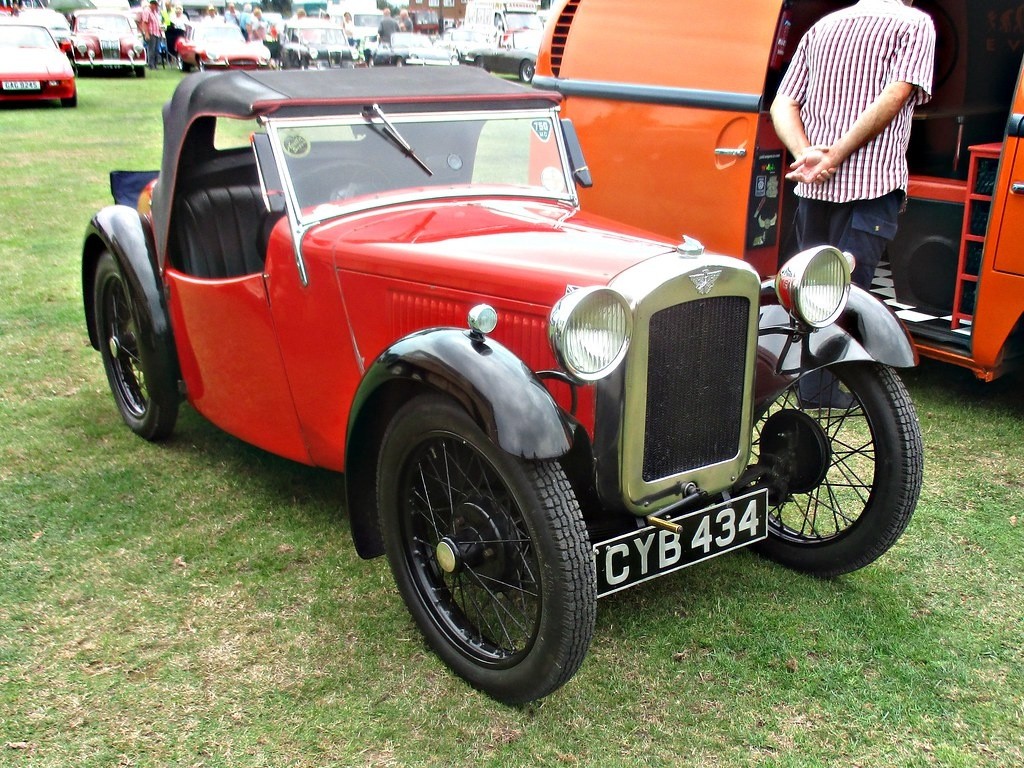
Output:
(883, 173), (968, 314)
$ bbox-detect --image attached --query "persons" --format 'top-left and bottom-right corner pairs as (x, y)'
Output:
(133, 0), (413, 71)
(770, 0), (937, 412)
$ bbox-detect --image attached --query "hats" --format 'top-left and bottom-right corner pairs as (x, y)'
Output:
(150, 0), (159, 4)
(209, 6), (214, 11)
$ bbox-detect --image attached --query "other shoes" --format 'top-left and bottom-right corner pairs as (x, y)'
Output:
(787, 383), (860, 411)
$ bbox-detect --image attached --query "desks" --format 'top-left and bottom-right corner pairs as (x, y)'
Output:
(913, 104), (1004, 178)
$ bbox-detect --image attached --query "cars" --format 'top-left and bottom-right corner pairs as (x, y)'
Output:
(372, 32), (463, 66)
(79, 63), (923, 709)
(66, 9), (147, 78)
(0, 17), (78, 108)
(18, 7), (71, 47)
(279, 21), (368, 71)
(435, 29), (539, 83)
(173, 21), (271, 72)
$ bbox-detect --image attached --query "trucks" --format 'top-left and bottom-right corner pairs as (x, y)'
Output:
(464, 0), (545, 51)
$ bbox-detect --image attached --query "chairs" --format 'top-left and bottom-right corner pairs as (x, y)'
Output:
(178, 152), (456, 280)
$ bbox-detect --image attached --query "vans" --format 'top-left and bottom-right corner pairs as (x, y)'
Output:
(317, 3), (385, 44)
